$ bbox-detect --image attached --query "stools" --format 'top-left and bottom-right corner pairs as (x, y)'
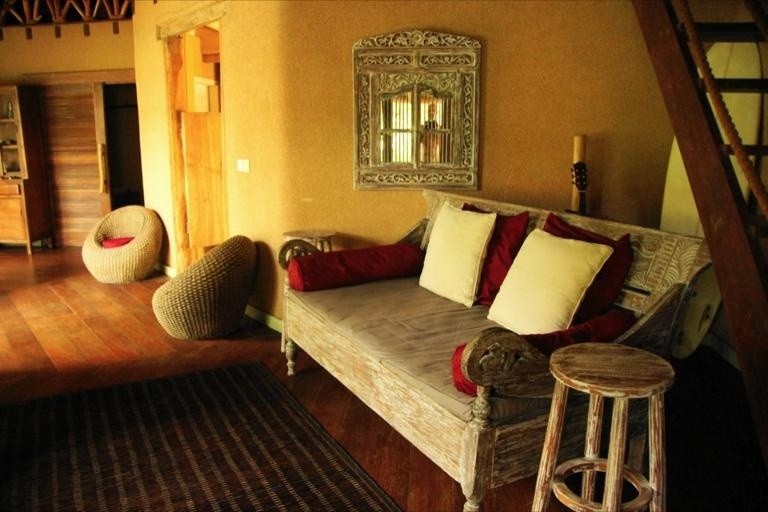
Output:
(532, 343), (676, 512)
(282, 232), (335, 351)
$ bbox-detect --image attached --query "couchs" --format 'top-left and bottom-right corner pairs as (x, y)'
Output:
(152, 235), (257, 340)
(82, 205), (163, 285)
(278, 190), (721, 512)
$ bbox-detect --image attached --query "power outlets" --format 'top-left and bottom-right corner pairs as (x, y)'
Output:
(237, 160), (250, 170)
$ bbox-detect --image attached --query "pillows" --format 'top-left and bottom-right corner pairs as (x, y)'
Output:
(452, 309), (636, 397)
(101, 237), (133, 248)
(288, 244), (424, 292)
(542, 213), (633, 324)
(487, 229), (614, 335)
(418, 203), (498, 308)
(462, 203), (529, 306)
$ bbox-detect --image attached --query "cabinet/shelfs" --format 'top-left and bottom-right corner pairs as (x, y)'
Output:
(0, 85), (48, 178)
(0, 179), (50, 254)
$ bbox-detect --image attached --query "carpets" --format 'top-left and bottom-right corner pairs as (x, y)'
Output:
(0, 362), (405, 512)
